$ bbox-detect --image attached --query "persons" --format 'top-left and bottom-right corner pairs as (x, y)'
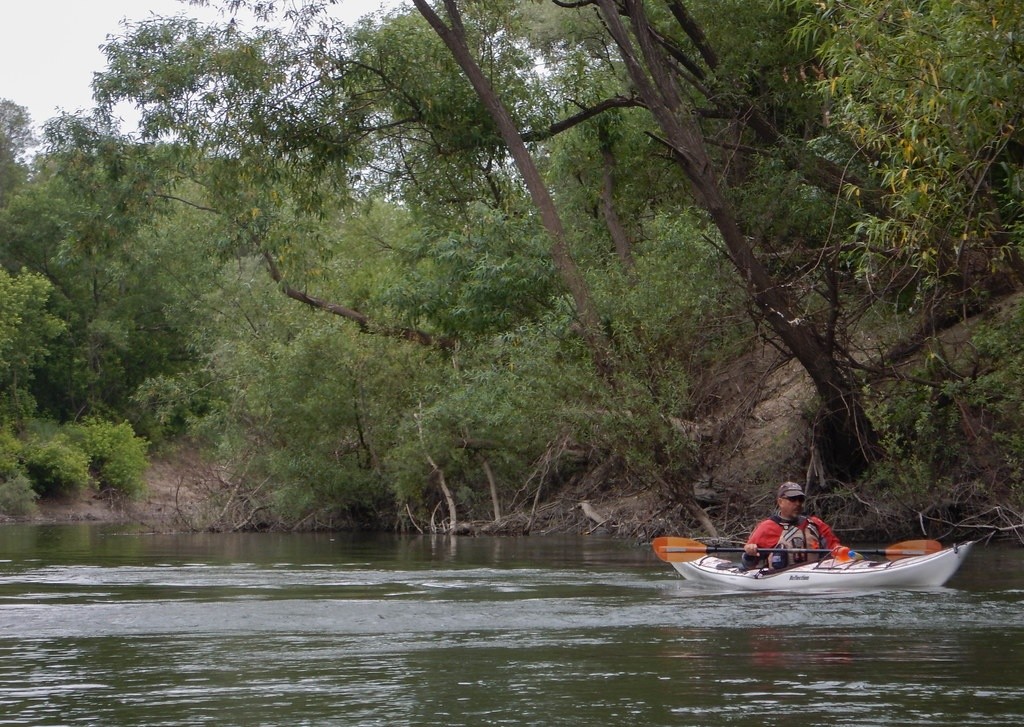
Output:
(742, 483), (843, 571)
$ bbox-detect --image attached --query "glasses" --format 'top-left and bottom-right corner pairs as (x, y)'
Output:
(782, 496), (805, 502)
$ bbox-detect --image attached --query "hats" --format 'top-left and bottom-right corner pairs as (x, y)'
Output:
(778, 482), (805, 498)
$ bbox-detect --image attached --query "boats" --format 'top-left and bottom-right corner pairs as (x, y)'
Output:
(670, 541), (976, 592)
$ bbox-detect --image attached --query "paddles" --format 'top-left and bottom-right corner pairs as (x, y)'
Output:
(652, 537), (942, 563)
